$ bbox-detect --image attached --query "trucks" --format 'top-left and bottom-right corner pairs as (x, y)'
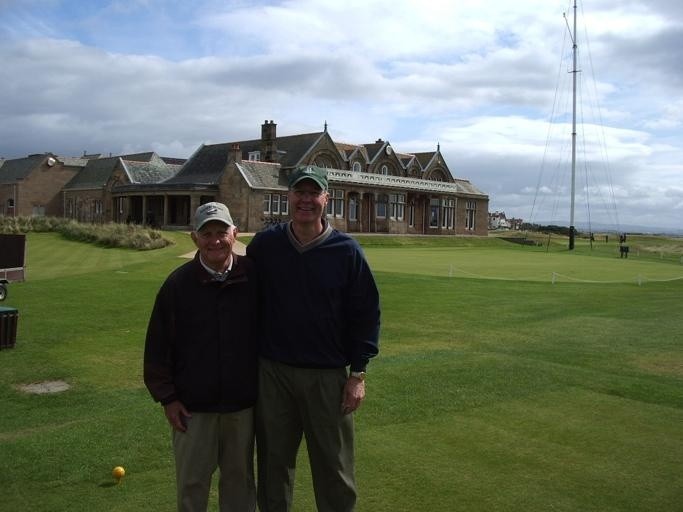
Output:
(0, 233), (27, 302)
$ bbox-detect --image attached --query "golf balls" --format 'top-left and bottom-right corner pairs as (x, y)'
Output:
(112, 466), (125, 479)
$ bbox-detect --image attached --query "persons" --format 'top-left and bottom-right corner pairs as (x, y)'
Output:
(246, 166), (380, 512)
(144, 202), (257, 512)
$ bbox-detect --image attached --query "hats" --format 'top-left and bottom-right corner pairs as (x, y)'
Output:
(194, 200), (234, 232)
(287, 165), (329, 192)
